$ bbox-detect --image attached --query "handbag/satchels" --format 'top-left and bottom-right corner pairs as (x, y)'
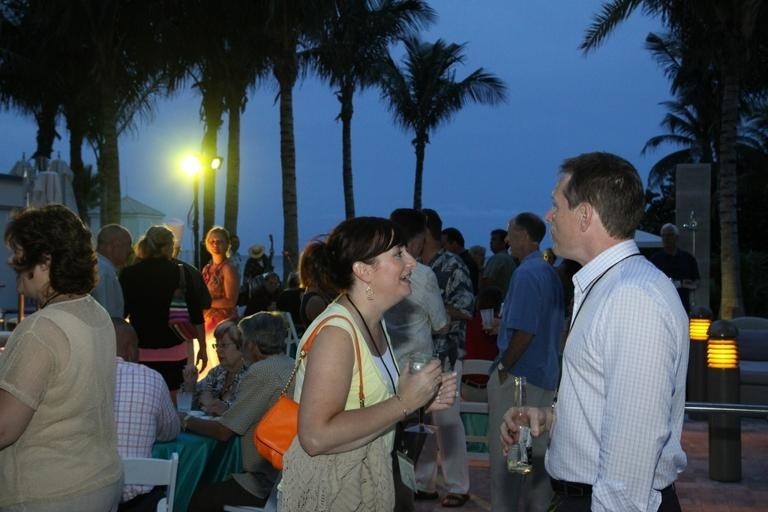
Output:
(252, 394), (310, 472)
(168, 263), (195, 344)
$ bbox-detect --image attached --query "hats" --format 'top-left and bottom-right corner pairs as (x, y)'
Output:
(248, 245), (265, 260)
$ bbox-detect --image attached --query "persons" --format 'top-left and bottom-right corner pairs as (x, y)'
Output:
(652, 222), (700, 318)
(0, 202), (128, 510)
(494, 150), (692, 511)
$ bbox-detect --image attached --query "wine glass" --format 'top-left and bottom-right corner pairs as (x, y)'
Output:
(404, 351), (440, 434)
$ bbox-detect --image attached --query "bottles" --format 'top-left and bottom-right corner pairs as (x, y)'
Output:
(505, 375), (534, 475)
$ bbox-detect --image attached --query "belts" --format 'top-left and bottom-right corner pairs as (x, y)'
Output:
(462, 379), (487, 389)
(549, 475), (676, 499)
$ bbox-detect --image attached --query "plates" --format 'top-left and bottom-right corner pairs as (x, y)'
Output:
(188, 409), (205, 418)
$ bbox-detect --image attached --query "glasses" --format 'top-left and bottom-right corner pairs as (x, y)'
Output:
(211, 342), (236, 350)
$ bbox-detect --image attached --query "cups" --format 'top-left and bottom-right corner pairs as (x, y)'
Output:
(176, 383), (193, 414)
(479, 308), (495, 335)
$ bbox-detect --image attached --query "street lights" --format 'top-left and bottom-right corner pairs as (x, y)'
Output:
(182, 152), (224, 267)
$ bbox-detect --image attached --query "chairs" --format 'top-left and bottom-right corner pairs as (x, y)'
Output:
(459, 358), (496, 462)
(115, 452), (181, 512)
(727, 315), (766, 406)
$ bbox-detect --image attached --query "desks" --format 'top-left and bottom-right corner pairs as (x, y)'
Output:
(146, 418), (242, 512)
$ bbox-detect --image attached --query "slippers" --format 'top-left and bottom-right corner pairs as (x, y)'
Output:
(439, 490), (471, 508)
(414, 489), (439, 501)
